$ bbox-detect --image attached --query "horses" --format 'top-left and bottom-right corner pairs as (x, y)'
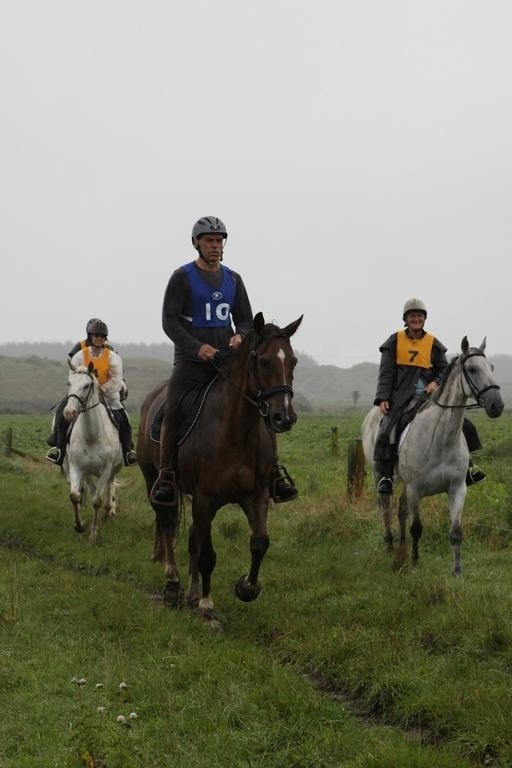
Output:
(51, 357), (131, 545)
(136, 312), (304, 611)
(360, 335), (504, 576)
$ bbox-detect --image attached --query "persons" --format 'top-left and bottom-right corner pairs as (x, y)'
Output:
(46, 321), (138, 464)
(152, 214), (299, 500)
(44, 317), (135, 449)
(374, 297), (487, 494)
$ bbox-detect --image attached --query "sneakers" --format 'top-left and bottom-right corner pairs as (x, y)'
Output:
(47, 447), (62, 464)
(377, 476), (392, 495)
(124, 448), (139, 467)
(152, 470), (176, 505)
(270, 467), (298, 501)
(465, 470), (486, 486)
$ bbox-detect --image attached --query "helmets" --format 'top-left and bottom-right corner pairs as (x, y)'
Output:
(402, 298), (426, 320)
(191, 216), (227, 243)
(85, 319), (107, 335)
